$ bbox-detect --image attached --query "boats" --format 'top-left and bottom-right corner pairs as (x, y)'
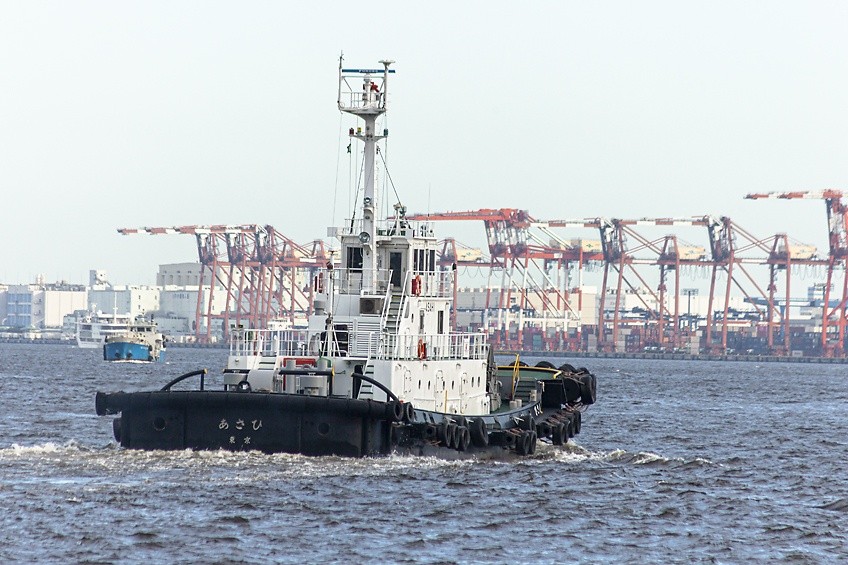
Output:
(103, 310), (168, 363)
(75, 302), (130, 348)
(94, 50), (597, 458)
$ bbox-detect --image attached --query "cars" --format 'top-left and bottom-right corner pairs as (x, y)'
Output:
(644, 345), (691, 354)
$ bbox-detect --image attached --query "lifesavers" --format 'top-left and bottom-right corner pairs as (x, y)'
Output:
(525, 416), (536, 432)
(387, 401), (404, 421)
(526, 430), (537, 454)
(567, 415), (576, 438)
(554, 423), (565, 445)
(450, 424), (460, 449)
(515, 432), (530, 456)
(472, 419), (488, 448)
(590, 374), (596, 402)
(403, 402), (414, 423)
(561, 419), (571, 443)
(509, 361), (589, 374)
(458, 425), (471, 451)
(418, 339), (425, 360)
(574, 411), (581, 434)
(457, 417), (468, 427)
(424, 425), (436, 439)
(437, 422), (451, 447)
(416, 276), (421, 295)
(580, 376), (595, 404)
